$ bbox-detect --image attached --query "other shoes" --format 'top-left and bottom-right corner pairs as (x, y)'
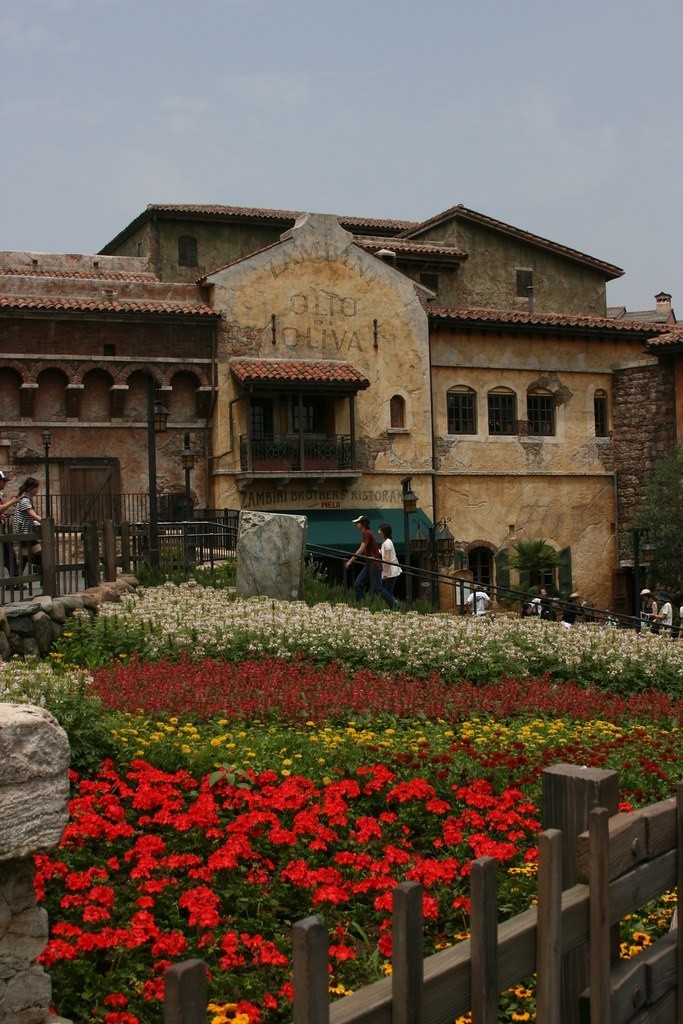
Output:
(5, 586), (16, 590)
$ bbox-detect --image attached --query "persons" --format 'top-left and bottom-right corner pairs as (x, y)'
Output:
(467, 584), (683, 639)
(345, 515), (403, 612)
(0, 470), (45, 591)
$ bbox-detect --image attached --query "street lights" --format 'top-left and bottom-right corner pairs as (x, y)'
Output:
(627, 524), (655, 633)
(39, 427), (53, 518)
(141, 365), (171, 572)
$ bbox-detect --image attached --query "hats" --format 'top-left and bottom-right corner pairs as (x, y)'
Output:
(640, 589), (651, 595)
(570, 593), (580, 598)
(477, 585), (481, 590)
(656, 591), (670, 601)
(481, 587), (487, 591)
(0, 471), (12, 482)
(352, 515), (370, 525)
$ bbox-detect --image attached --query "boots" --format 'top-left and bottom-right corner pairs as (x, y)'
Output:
(16, 555), (28, 590)
(32, 551), (44, 589)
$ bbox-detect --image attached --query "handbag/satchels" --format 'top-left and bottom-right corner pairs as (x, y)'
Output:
(640, 612), (649, 628)
